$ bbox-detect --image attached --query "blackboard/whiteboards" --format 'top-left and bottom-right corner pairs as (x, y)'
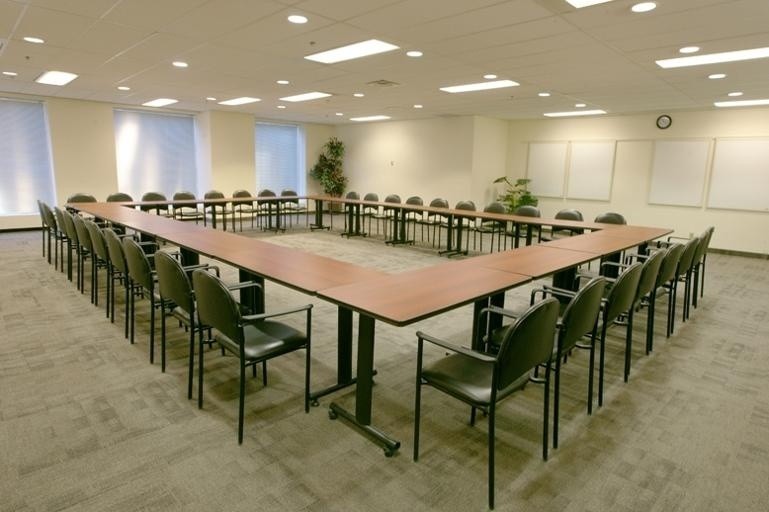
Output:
(647, 136), (769, 212)
(525, 140), (618, 203)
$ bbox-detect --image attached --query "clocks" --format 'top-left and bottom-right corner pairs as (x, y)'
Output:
(656, 114), (672, 129)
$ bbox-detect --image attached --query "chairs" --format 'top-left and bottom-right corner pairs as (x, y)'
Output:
(36, 190), (629, 258)
(412, 296), (561, 511)
(481, 274), (607, 449)
(470, 226), (714, 407)
(155, 250), (257, 401)
(192, 267), (314, 445)
(36, 198), (207, 364)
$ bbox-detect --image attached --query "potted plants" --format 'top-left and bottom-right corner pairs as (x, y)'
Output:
(308, 136), (347, 215)
(494, 177), (538, 233)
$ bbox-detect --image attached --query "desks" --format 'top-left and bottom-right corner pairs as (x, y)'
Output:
(316, 259), (534, 458)
(157, 229), (378, 407)
